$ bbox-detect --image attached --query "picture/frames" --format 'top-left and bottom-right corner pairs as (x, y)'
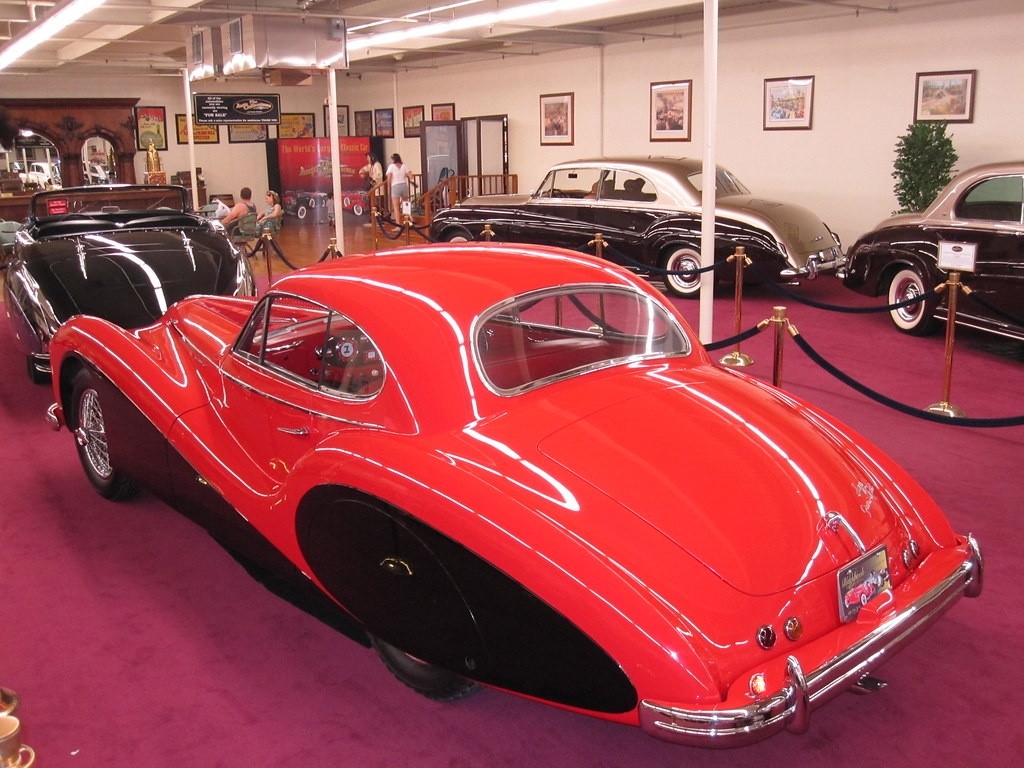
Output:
(228, 124), (269, 143)
(913, 69), (977, 124)
(431, 102), (455, 122)
(540, 91), (575, 146)
(649, 79), (692, 142)
(763, 75), (815, 130)
(375, 108), (394, 138)
(403, 104), (425, 138)
(277, 112), (316, 139)
(175, 114), (220, 144)
(134, 106), (168, 151)
(323, 104), (349, 137)
(354, 110), (372, 137)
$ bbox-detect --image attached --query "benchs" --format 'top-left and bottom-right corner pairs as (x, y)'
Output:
(358, 335), (610, 398)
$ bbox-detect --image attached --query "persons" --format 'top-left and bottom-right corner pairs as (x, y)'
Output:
(386, 153), (413, 231)
(359, 152), (384, 208)
(221, 187), (256, 236)
(256, 191), (281, 231)
(656, 111), (683, 130)
(545, 116), (563, 135)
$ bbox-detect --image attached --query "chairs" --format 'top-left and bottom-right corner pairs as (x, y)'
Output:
(583, 178), (645, 199)
(199, 204), (285, 259)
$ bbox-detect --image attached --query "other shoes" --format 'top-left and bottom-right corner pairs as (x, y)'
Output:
(391, 227), (400, 232)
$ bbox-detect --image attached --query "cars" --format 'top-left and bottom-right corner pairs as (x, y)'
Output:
(427, 156), (846, 299)
(839, 163), (1024, 345)
(283, 189), (327, 219)
(45, 240), (986, 749)
(341, 190), (371, 215)
(0, 183), (256, 385)
